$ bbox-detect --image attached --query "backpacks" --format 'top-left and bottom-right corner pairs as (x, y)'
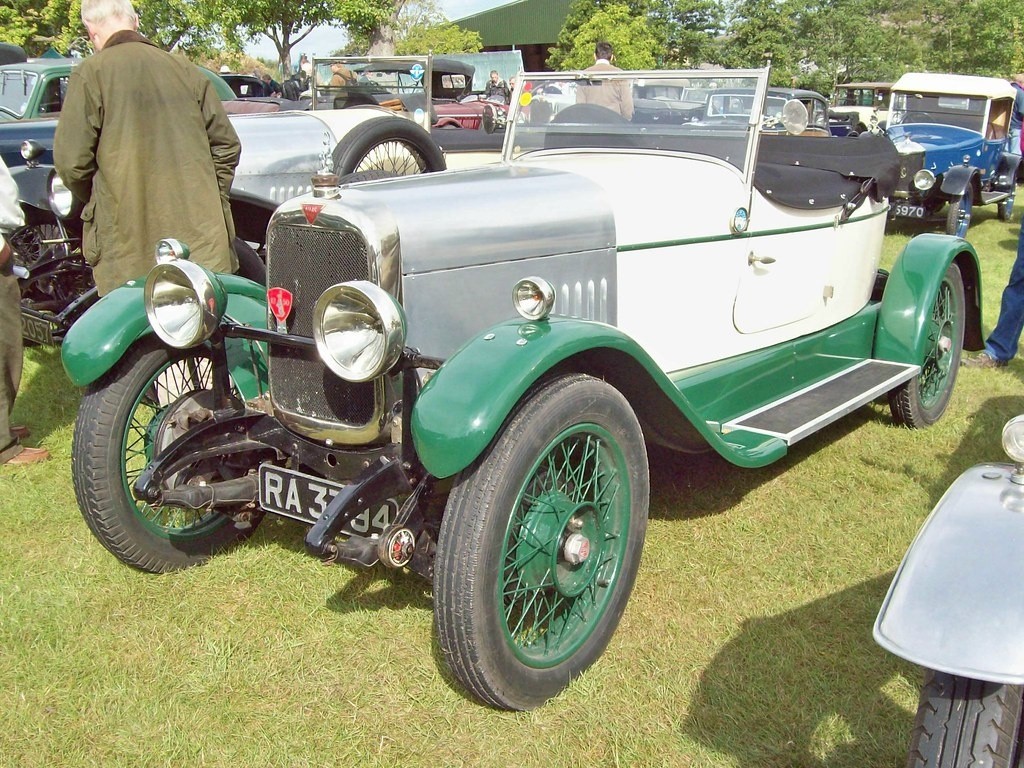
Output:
(333, 71), (359, 87)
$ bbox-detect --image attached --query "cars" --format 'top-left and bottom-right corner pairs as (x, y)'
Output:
(7, 50), (545, 348)
(829, 82), (894, 136)
(352, 59), (529, 131)
(530, 78), (708, 125)
(62, 60), (998, 713)
(879, 73), (1023, 238)
(681, 87), (832, 138)
(0, 65), (238, 167)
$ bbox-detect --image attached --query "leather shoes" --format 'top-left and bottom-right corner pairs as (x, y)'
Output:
(5, 447), (52, 465)
(8, 424), (31, 439)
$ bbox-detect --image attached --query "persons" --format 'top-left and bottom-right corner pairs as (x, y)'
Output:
(263, 74), (282, 99)
(531, 88), (553, 124)
(0, 153), (52, 466)
(576, 42), (637, 121)
(846, 89), (856, 105)
(485, 70), (510, 105)
(220, 65), (231, 75)
(53, 0), (242, 510)
(508, 78), (516, 105)
(318, 60), (358, 96)
(960, 65), (1024, 369)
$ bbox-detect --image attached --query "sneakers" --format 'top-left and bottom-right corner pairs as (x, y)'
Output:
(959, 352), (1009, 369)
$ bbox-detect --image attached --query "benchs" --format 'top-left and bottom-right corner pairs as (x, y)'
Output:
(544, 101), (902, 214)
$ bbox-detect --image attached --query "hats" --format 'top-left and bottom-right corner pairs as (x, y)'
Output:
(218, 65), (231, 73)
(323, 59), (340, 67)
